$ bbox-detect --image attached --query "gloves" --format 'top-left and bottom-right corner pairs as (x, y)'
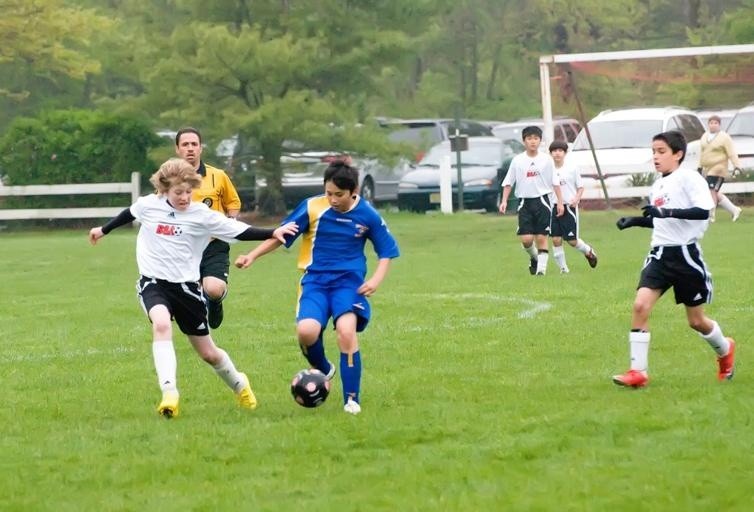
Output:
(641, 205), (666, 218)
(617, 217), (633, 230)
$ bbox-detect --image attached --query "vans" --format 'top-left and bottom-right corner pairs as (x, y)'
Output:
(561, 104), (754, 205)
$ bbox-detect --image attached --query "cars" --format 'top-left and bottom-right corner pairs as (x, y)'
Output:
(154, 118), (399, 202)
(402, 117), (576, 137)
(399, 137), (526, 211)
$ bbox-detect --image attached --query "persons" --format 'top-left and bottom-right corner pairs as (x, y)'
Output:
(546, 140), (598, 274)
(697, 114), (744, 223)
(497, 125), (565, 276)
(234, 160), (401, 416)
(88, 157), (299, 417)
(613, 129), (735, 388)
(153, 127), (242, 330)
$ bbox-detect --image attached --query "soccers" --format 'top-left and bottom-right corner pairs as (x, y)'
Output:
(288, 367), (332, 408)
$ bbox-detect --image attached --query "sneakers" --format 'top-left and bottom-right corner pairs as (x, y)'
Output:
(585, 246), (597, 267)
(613, 371), (648, 387)
(717, 337), (735, 380)
(710, 207), (742, 223)
(158, 391), (179, 417)
(529, 256), (570, 276)
(326, 360), (336, 380)
(232, 372), (257, 410)
(344, 401), (361, 414)
(205, 299), (223, 329)
